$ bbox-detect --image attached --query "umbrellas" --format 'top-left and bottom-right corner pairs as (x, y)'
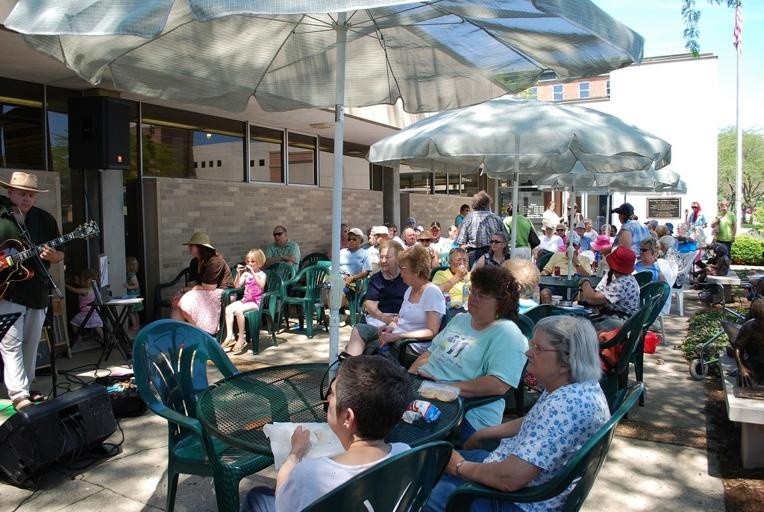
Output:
(2, 0), (645, 417)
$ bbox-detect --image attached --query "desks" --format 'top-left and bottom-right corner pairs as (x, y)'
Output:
(88, 297), (145, 368)
(197, 360), (462, 511)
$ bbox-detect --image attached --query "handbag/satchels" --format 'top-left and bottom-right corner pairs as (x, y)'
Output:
(528, 227), (540, 249)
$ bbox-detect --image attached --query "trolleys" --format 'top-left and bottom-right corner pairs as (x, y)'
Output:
(689, 281), (750, 381)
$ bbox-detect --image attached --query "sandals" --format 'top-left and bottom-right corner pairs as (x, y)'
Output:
(29, 391), (48, 402)
(221, 333), (237, 349)
(232, 342), (248, 355)
(12, 396), (35, 411)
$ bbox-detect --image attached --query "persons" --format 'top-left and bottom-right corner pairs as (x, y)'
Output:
(1, 172), (65, 412)
(65, 193), (763, 510)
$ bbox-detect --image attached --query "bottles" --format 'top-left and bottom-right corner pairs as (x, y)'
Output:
(444, 290), (451, 309)
(462, 280), (469, 304)
(534, 283), (541, 304)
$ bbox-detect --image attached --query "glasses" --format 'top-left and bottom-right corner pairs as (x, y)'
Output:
(528, 339), (570, 355)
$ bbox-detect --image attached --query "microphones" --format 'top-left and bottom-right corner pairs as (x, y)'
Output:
(8, 208), (19, 225)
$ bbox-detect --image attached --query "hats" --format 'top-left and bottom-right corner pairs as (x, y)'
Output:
(716, 244), (727, 255)
(347, 217), (441, 239)
(541, 203), (673, 273)
(0, 172), (50, 193)
(182, 232), (216, 250)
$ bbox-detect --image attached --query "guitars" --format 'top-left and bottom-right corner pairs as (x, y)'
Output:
(1, 219), (104, 298)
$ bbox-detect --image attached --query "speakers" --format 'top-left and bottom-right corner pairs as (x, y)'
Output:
(0, 383), (119, 488)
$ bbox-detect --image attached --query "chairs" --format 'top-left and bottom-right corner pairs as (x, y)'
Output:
(445, 384), (644, 512)
(131, 319), (289, 512)
(295, 439), (453, 512)
